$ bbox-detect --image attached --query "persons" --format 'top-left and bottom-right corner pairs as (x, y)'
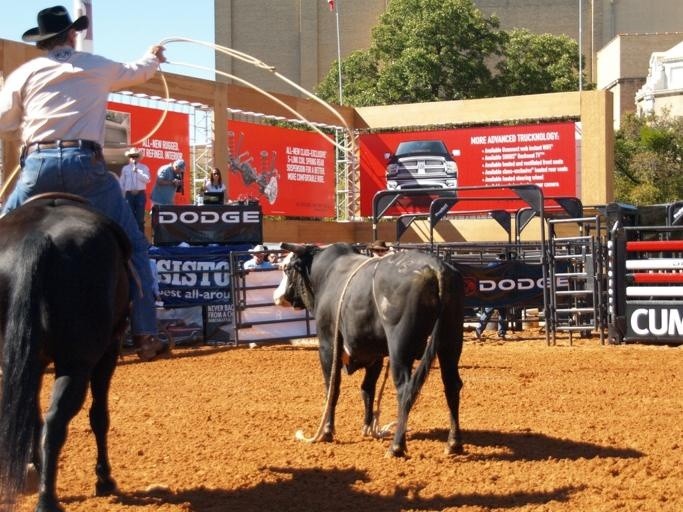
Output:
(370, 241), (389, 258)
(268, 251), (277, 263)
(118, 147), (151, 235)
(0, 5), (174, 362)
(202, 168), (228, 204)
(242, 245), (274, 268)
(150, 159), (184, 213)
(475, 253), (509, 338)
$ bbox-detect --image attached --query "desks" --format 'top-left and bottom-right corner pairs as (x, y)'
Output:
(150, 204), (261, 249)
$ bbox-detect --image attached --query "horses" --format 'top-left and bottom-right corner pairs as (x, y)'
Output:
(0, 194), (132, 512)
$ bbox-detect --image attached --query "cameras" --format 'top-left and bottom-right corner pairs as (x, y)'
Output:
(176, 174), (185, 194)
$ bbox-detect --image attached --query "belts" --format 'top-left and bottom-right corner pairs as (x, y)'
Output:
(24, 140), (101, 156)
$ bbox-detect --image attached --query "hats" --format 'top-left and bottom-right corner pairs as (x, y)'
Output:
(367, 240), (389, 250)
(248, 245), (267, 255)
(177, 160), (185, 170)
(124, 148), (144, 159)
(22, 5), (89, 43)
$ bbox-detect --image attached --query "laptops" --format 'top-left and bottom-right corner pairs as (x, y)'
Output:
(204, 191), (224, 204)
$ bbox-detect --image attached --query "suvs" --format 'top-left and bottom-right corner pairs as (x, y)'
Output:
(384, 139), (462, 207)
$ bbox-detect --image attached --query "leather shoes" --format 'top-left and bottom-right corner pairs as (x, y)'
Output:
(137, 335), (168, 360)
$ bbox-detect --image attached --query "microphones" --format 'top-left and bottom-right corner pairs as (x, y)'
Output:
(133, 160), (137, 172)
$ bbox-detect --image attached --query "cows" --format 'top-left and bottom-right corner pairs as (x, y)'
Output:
(272, 240), (467, 457)
